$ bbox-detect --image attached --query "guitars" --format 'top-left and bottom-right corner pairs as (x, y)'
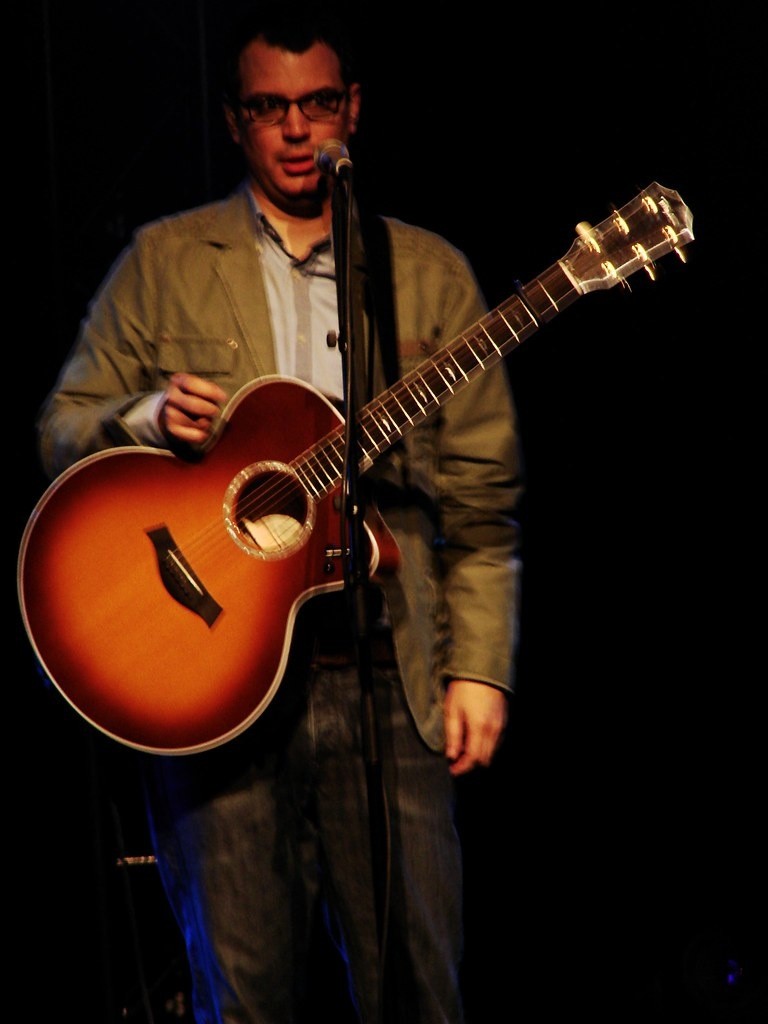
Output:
(16, 182), (696, 759)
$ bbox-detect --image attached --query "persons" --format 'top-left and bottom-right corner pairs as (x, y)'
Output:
(40, 1), (522, 1024)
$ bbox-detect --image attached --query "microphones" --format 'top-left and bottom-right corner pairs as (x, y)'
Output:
(314, 139), (354, 181)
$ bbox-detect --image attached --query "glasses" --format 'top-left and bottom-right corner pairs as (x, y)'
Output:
(236, 87), (347, 122)
